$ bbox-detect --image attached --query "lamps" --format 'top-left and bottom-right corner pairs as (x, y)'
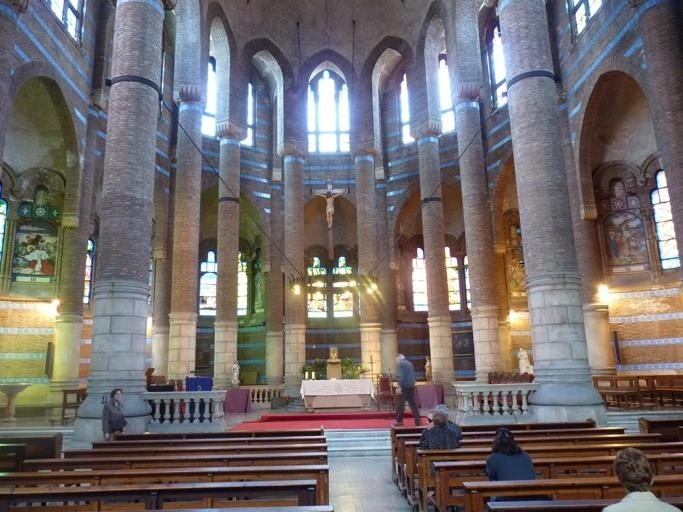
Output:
(158, 96), (506, 305)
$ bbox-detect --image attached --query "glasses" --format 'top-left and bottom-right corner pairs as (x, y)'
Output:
(428, 418), (434, 423)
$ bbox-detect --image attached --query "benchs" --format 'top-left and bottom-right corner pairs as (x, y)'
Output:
(387, 416), (683, 512)
(1, 426), (331, 512)
(592, 369), (682, 409)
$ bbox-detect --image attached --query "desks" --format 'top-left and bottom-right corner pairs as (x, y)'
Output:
(391, 380), (443, 410)
(299, 379), (371, 412)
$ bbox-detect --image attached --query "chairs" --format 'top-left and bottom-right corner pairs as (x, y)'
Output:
(57, 372), (185, 421)
(376, 371), (392, 408)
(470, 371), (535, 414)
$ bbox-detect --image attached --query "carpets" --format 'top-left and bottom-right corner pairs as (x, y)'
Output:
(228, 410), (431, 430)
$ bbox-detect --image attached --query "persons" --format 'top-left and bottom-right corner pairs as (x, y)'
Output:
(392, 353), (422, 427)
(102, 388), (128, 441)
(602, 447), (680, 512)
(419, 411), (459, 451)
(427, 405), (464, 439)
(486, 429), (536, 481)
